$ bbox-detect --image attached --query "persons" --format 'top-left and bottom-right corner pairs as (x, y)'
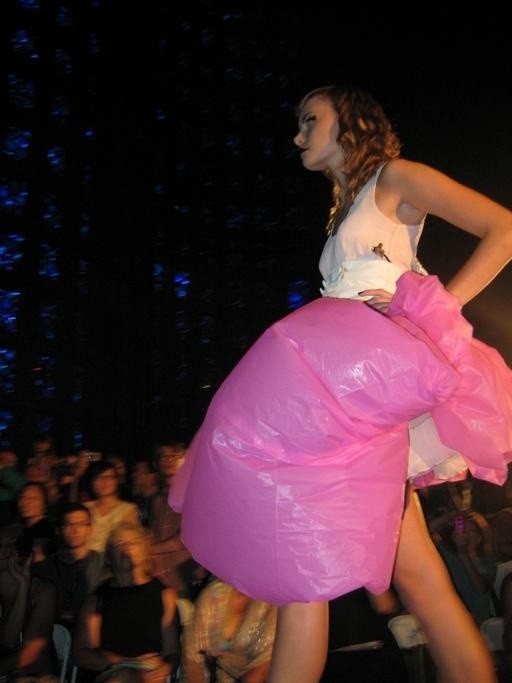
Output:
(367, 481), (511, 618)
(0, 441), (278, 683)
(166, 71), (511, 683)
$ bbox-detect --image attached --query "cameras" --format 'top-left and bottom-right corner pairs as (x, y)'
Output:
(83, 452), (102, 462)
